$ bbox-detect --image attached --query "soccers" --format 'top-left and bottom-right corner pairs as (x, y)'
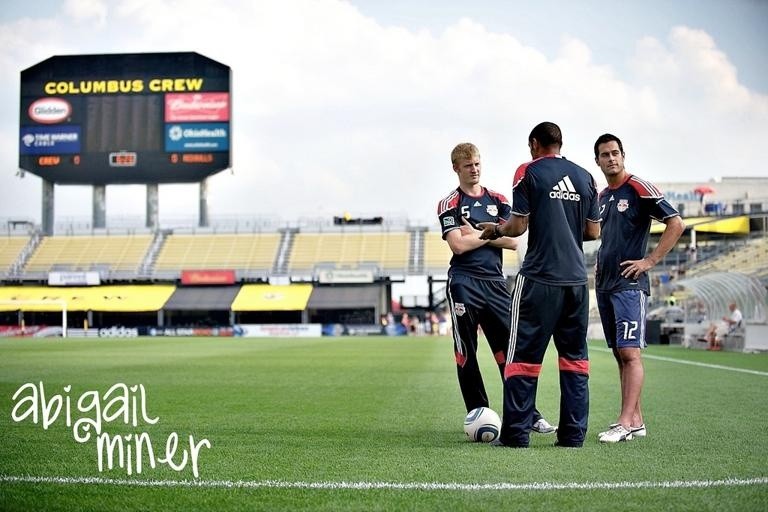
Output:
(463, 407), (502, 442)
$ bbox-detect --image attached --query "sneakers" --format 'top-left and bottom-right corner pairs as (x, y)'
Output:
(534, 418), (558, 435)
(490, 436), (531, 449)
(552, 438), (585, 448)
(602, 427), (631, 445)
(600, 422), (649, 437)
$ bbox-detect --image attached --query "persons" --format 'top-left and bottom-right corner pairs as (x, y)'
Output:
(594, 134), (686, 442)
(402, 311), (439, 335)
(479, 122), (602, 447)
(437, 144), (558, 433)
(697, 303), (742, 350)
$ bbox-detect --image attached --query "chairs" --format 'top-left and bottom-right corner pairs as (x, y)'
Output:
(723, 321), (745, 351)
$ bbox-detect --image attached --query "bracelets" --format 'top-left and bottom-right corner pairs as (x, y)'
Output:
(494, 225), (502, 237)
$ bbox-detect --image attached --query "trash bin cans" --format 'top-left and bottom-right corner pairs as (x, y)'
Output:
(645, 320), (661, 344)
